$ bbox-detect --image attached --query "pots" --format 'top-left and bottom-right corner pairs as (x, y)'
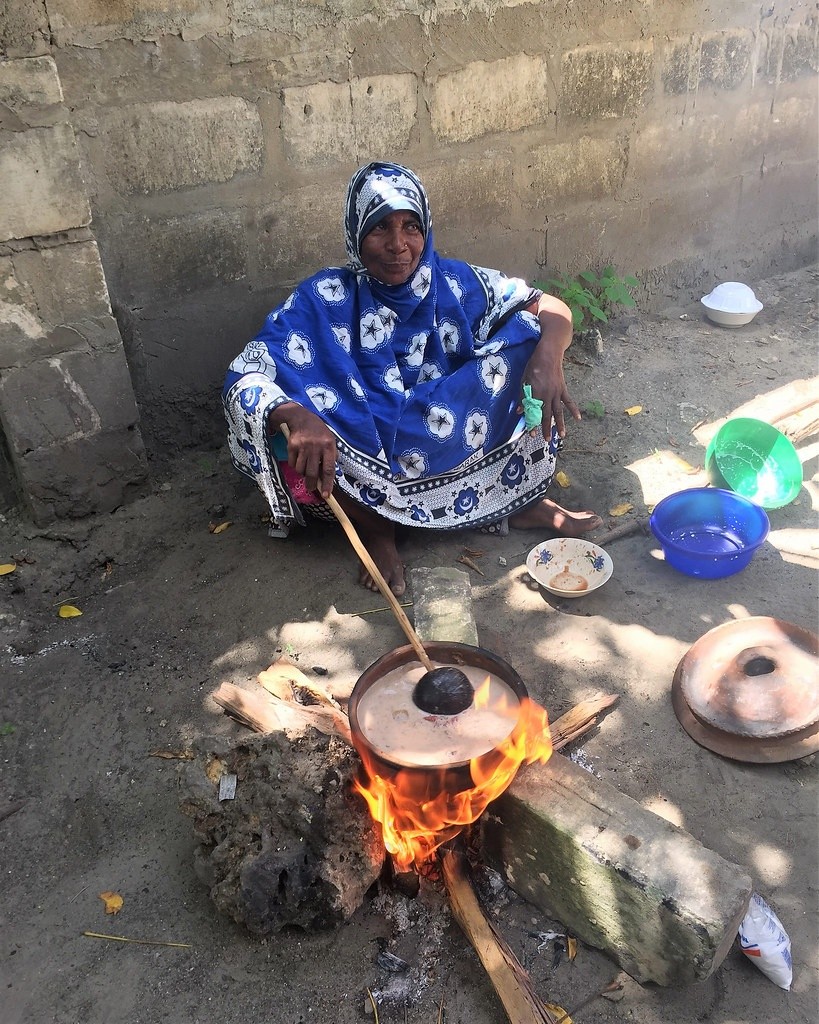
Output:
(346, 641), (530, 800)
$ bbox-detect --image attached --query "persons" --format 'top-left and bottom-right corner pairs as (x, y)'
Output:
(214, 161), (601, 597)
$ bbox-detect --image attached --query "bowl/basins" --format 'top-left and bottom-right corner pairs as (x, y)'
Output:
(701, 281), (764, 329)
(526, 538), (614, 598)
(648, 488), (771, 580)
(704, 418), (805, 511)
